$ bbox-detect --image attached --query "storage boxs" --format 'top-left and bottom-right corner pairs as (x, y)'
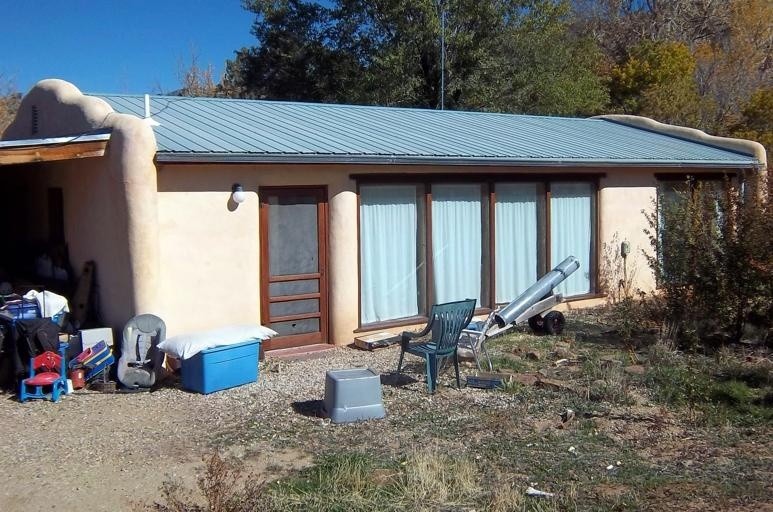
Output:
(178, 338), (264, 398)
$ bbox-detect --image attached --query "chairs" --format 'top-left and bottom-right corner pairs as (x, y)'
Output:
(20, 351), (68, 403)
(395, 298), (477, 397)
